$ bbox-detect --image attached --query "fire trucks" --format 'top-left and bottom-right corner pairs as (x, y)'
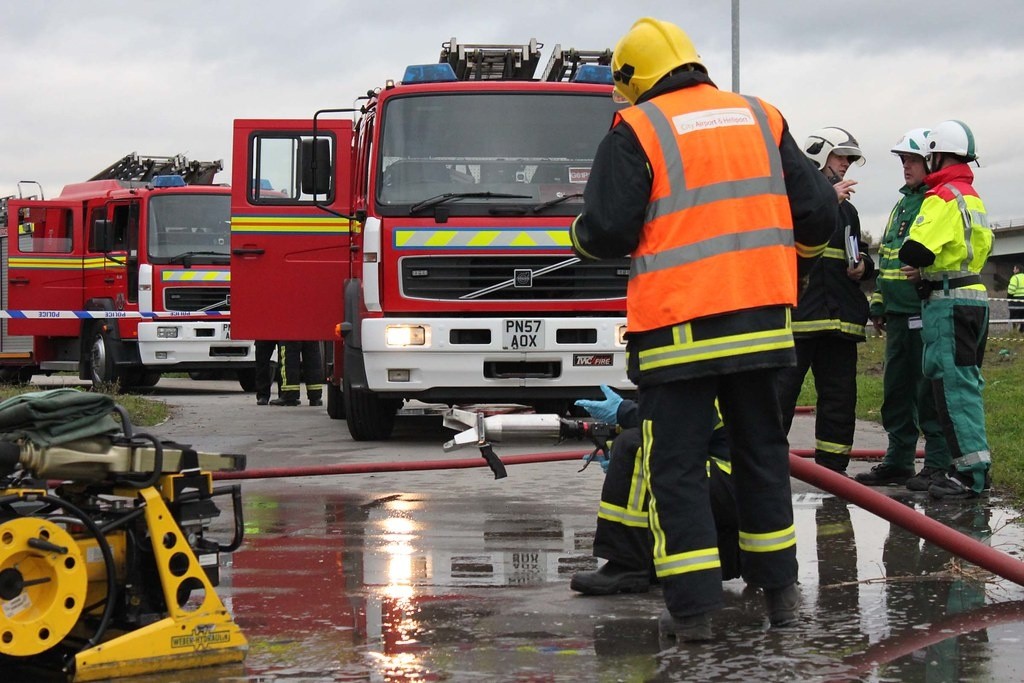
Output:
(232, 43), (635, 442)
(0, 153), (280, 392)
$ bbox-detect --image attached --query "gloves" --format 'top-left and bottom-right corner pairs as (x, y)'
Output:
(574, 382), (622, 426)
(582, 449), (611, 474)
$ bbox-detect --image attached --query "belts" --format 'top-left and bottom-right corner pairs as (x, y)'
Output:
(930, 275), (981, 290)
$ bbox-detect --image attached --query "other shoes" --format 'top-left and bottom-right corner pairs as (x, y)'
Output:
(309, 399), (323, 407)
(270, 397), (299, 407)
(257, 397), (267, 404)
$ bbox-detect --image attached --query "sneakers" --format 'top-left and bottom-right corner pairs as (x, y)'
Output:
(668, 613), (709, 641)
(855, 457), (914, 486)
(907, 465), (943, 490)
(764, 582), (801, 627)
(927, 469), (991, 498)
(569, 557), (658, 597)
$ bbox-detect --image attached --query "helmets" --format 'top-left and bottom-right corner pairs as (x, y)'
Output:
(922, 120), (975, 161)
(890, 130), (936, 173)
(803, 126), (865, 172)
(609, 18), (707, 106)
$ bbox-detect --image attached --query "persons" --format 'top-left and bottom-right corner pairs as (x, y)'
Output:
(1006, 264), (1024, 335)
(251, 339), (324, 407)
(570, 381), (786, 596)
(898, 115), (995, 497)
(781, 123), (877, 483)
(865, 287), (886, 336)
(570, 15), (840, 643)
(856, 128), (953, 490)
